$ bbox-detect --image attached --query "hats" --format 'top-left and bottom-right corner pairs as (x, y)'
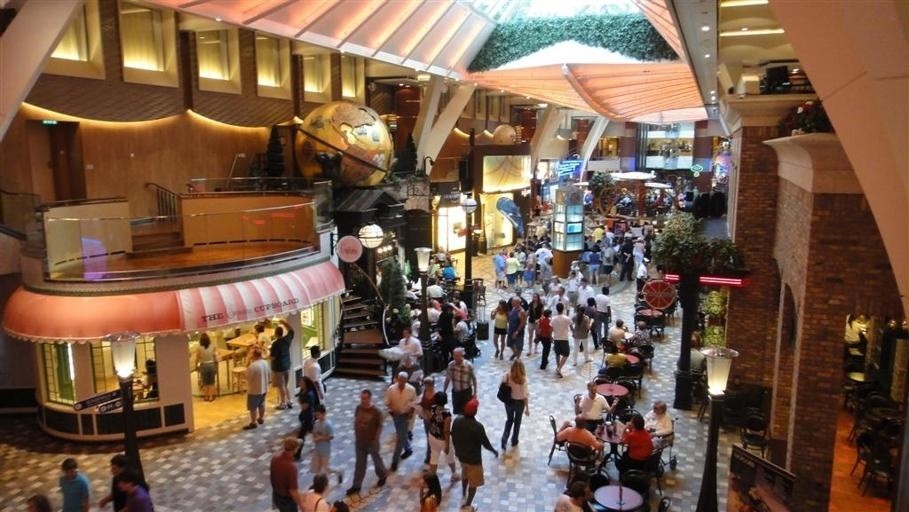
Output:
(465, 399), (478, 415)
(284, 436), (304, 449)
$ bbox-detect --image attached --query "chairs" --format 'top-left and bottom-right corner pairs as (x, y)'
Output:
(696, 379), (768, 459)
(843, 361), (904, 497)
(548, 278), (680, 512)
(406, 275), (486, 363)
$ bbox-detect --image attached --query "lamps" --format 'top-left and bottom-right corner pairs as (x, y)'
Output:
(359, 224), (384, 249)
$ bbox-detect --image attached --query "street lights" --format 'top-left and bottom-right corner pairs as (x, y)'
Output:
(414, 190), (480, 373)
(696, 346), (738, 510)
(112, 334), (144, 483)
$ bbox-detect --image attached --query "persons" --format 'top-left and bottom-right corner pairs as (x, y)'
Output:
(493, 175), (702, 291)
(27, 454), (155, 512)
(245, 259), (671, 512)
(138, 358), (158, 399)
(195, 332), (217, 402)
(844, 315), (871, 360)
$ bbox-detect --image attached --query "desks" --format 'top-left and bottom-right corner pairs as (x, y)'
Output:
(189, 334), (258, 397)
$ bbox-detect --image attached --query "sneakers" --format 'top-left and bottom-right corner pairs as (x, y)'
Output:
(501, 438), (519, 449)
(307, 448), (414, 494)
(422, 462), (479, 511)
(495, 350), (594, 377)
(242, 400), (292, 430)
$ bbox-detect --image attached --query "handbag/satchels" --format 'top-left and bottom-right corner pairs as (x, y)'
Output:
(497, 382), (512, 406)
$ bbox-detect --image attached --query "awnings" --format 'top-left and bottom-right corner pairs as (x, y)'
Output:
(0, 260), (345, 346)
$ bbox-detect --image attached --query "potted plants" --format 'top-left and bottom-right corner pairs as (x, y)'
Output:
(705, 290), (725, 326)
(690, 237), (737, 269)
